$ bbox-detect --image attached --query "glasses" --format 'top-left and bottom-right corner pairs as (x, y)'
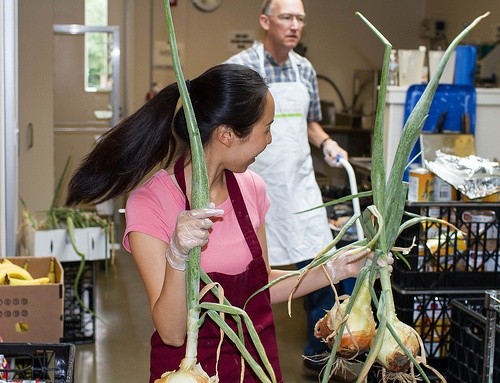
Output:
(268, 12), (307, 27)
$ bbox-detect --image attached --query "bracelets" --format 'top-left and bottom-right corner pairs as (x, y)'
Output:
(319, 137), (331, 152)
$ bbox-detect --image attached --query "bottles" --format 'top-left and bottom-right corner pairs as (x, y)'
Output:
(388, 50), (398, 86)
(419, 46), (428, 84)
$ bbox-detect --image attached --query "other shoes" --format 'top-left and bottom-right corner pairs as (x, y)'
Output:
(303, 355), (326, 374)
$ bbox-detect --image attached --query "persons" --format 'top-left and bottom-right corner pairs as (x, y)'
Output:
(64, 64), (395, 383)
(223, 0), (350, 268)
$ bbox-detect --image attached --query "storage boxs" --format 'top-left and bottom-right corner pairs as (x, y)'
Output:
(0, 256), (65, 342)
(62, 263), (96, 345)
(24, 211), (110, 262)
(0, 343), (75, 383)
(391, 201), (500, 383)
